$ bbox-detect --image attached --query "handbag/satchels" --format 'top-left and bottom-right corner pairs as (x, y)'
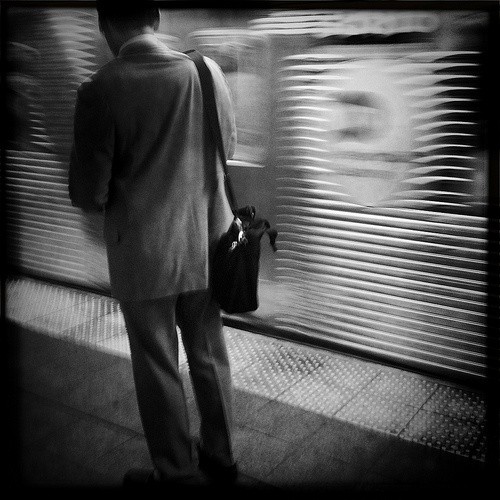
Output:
(208, 202), (277, 316)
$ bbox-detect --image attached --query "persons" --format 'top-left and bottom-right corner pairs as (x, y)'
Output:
(64, 1), (240, 492)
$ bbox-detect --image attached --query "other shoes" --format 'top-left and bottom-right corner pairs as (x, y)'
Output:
(192, 435), (240, 481)
(124, 468), (193, 488)
